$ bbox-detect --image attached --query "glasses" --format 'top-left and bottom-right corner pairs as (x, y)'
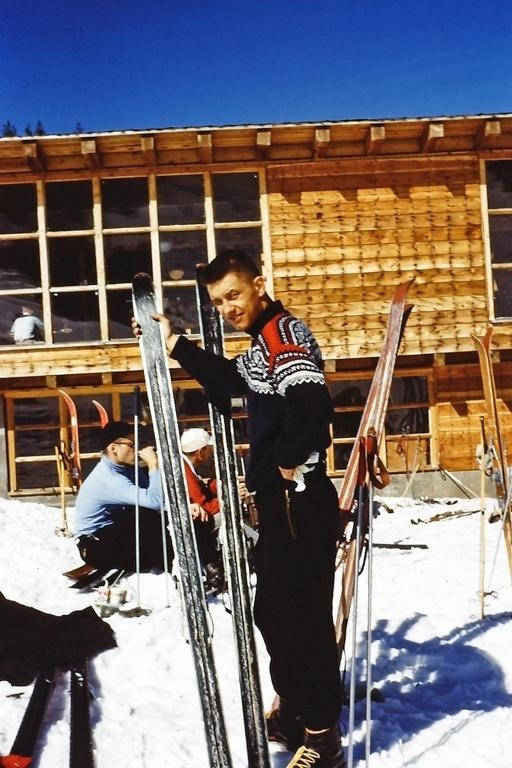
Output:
(112, 442), (135, 448)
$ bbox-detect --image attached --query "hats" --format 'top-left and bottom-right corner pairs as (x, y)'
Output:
(181, 428), (214, 453)
(91, 421), (134, 450)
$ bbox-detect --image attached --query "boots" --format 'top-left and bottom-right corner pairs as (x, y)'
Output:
(265, 694), (345, 768)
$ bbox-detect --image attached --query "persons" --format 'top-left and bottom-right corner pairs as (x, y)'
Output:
(72, 421), (175, 573)
(132, 250), (347, 768)
(181, 428), (246, 596)
(11, 307), (45, 345)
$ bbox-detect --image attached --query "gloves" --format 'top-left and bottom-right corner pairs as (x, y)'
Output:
(292, 448), (320, 492)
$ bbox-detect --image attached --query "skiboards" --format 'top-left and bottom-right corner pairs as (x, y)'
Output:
(333, 277), (417, 705)
(469, 327), (511, 583)
(59, 389), (109, 495)
(130, 265), (270, 768)
(2, 628), (95, 768)
